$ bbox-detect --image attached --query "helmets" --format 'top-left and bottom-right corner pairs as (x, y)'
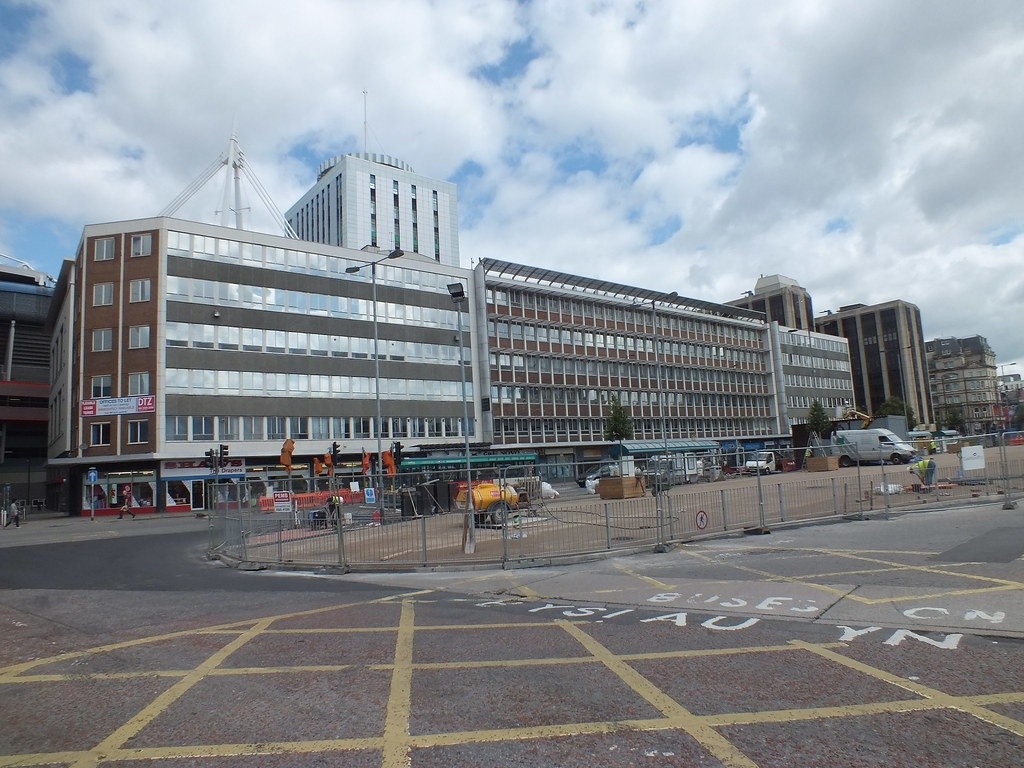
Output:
(907, 466), (911, 472)
(931, 440), (934, 442)
(810, 446), (812, 448)
(340, 497), (343, 504)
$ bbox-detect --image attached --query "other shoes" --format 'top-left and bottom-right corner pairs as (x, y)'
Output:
(16, 527), (21, 528)
(923, 489), (931, 493)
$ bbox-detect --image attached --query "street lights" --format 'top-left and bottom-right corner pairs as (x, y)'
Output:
(445, 281), (476, 550)
(784, 317), (832, 411)
(878, 344), (915, 431)
(628, 292), (679, 484)
(1000, 361), (1017, 428)
(345, 249), (405, 518)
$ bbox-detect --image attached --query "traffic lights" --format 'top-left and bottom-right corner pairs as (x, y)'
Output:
(396, 441), (405, 465)
(333, 442), (340, 466)
(205, 449), (214, 468)
(219, 444), (229, 469)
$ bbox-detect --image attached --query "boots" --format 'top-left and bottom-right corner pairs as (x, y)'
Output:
(117, 510), (123, 519)
(128, 510), (136, 518)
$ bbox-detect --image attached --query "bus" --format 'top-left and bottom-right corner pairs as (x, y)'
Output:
(396, 452), (542, 510)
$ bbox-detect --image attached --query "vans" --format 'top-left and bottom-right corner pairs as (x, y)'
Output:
(747, 452), (776, 475)
(830, 428), (917, 468)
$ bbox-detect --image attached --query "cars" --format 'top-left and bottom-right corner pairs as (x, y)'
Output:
(575, 458), (618, 488)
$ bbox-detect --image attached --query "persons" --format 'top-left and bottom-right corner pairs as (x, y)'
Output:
(907, 460), (936, 489)
(5, 498), (21, 529)
(117, 490), (136, 520)
(326, 496), (341, 529)
(929, 440), (937, 454)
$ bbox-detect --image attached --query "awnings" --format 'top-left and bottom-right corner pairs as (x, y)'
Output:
(160, 459), (246, 481)
